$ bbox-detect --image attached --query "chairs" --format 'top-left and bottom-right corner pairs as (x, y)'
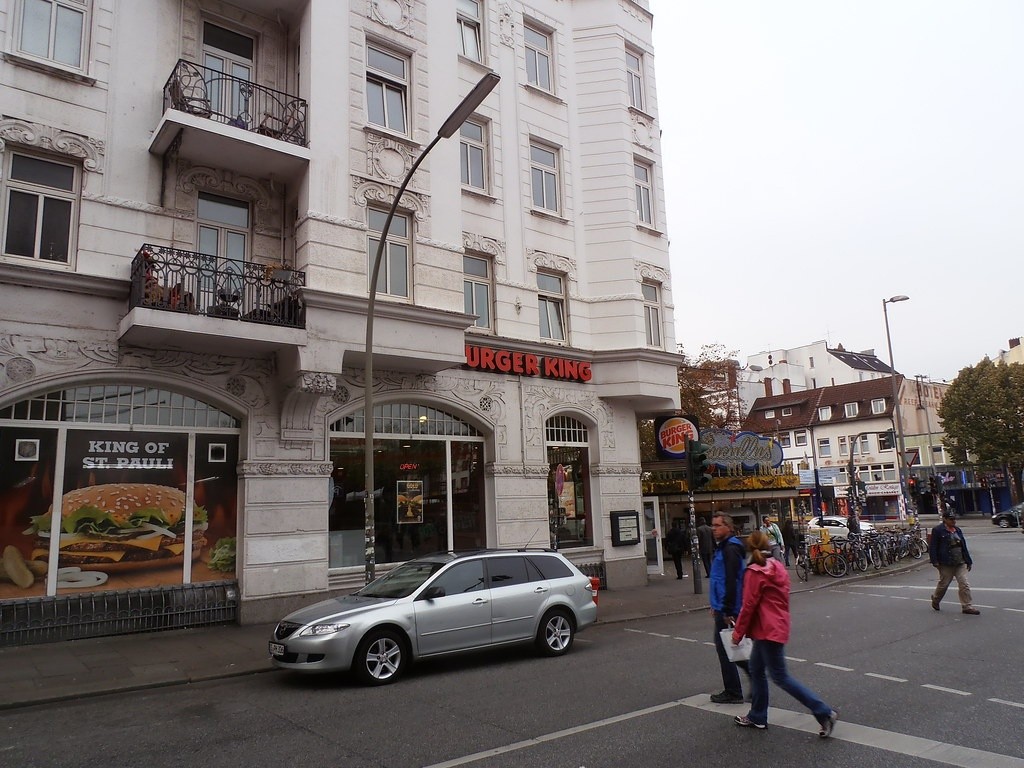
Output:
(168, 78), (213, 119)
(134, 251), (195, 314)
(256, 109), (295, 138)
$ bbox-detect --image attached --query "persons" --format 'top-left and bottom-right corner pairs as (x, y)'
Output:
(732, 531), (838, 738)
(664, 521), (687, 580)
(695, 516), (717, 578)
(709, 511), (754, 703)
(759, 515), (785, 561)
(782, 519), (800, 566)
(929, 510), (980, 615)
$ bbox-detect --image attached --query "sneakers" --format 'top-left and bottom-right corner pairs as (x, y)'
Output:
(734, 712), (767, 730)
(818, 709), (837, 737)
(711, 689), (744, 703)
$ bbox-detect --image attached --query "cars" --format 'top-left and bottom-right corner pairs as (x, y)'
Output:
(991, 502), (1024, 528)
(268, 547), (599, 686)
(807, 516), (876, 536)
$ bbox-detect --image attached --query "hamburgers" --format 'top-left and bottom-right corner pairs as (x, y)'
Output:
(21, 483), (210, 572)
(397, 495), (423, 520)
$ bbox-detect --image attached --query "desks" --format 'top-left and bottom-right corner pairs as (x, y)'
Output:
(216, 289), (241, 319)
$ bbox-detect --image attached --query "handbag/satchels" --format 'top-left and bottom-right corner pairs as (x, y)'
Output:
(719, 619), (753, 663)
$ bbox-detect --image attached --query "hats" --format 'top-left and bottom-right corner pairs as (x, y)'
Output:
(943, 510), (957, 519)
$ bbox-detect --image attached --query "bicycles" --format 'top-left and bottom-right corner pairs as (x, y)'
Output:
(796, 523), (929, 582)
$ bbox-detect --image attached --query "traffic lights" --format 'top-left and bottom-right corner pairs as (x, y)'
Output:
(858, 481), (867, 496)
(908, 478), (917, 497)
(981, 477), (987, 488)
(690, 441), (710, 490)
(928, 475), (939, 494)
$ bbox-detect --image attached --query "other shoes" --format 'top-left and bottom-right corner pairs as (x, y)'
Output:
(705, 574), (709, 577)
(932, 594), (941, 611)
(962, 607), (980, 615)
(677, 575), (682, 579)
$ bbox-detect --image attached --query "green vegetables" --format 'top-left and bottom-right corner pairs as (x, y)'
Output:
(208, 536), (237, 572)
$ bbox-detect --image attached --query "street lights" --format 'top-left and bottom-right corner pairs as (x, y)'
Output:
(882, 295), (910, 518)
(365, 71), (500, 585)
(884, 428), (895, 448)
